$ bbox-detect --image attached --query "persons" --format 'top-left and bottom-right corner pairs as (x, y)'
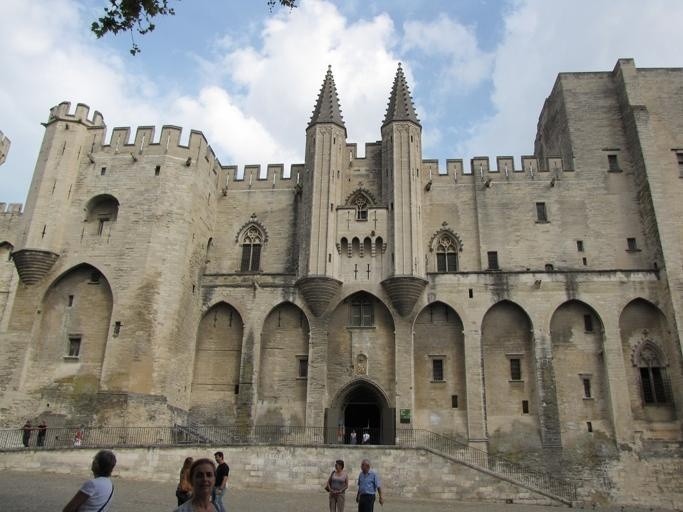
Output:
(73, 423), (84, 447)
(355, 458), (384, 512)
(174, 456), (193, 507)
(349, 428), (358, 444)
(33, 419), (47, 446)
(326, 460), (349, 511)
(20, 419), (32, 447)
(362, 430), (370, 444)
(211, 452), (229, 512)
(171, 457), (225, 511)
(61, 448), (117, 511)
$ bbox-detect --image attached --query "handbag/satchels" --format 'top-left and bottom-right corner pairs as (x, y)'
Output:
(324, 471), (334, 492)
(176, 491), (188, 500)
